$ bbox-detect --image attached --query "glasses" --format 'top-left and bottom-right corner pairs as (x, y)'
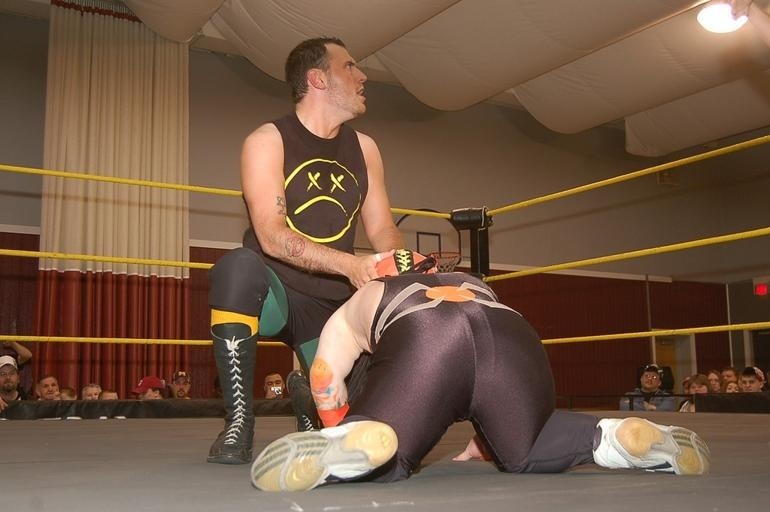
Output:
(641, 373), (660, 381)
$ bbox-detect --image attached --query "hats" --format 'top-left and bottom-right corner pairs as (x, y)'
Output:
(642, 363), (664, 379)
(172, 370), (193, 385)
(0, 355), (19, 371)
(131, 376), (166, 394)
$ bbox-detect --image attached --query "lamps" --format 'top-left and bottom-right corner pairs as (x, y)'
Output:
(696, 1), (751, 35)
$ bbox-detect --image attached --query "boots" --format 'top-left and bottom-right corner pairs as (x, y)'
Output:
(287, 368), (317, 433)
(201, 322), (262, 466)
(590, 410), (719, 480)
(249, 410), (400, 495)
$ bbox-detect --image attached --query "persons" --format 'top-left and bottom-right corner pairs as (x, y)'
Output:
(0, 337), (193, 420)
(262, 369), (285, 400)
(202, 34), (439, 466)
(618, 363), (765, 414)
(246, 272), (714, 496)
(214, 377), (223, 398)
